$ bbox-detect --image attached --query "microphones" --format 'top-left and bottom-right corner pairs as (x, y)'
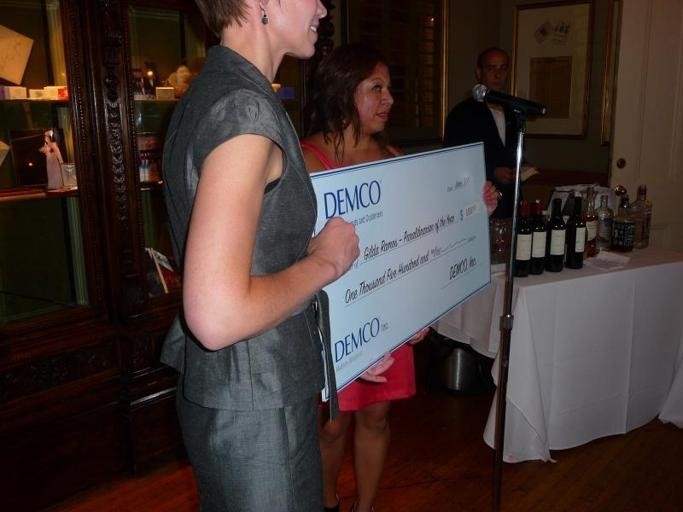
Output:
(470, 84), (547, 118)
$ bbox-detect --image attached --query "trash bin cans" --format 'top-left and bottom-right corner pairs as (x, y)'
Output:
(439, 347), (478, 394)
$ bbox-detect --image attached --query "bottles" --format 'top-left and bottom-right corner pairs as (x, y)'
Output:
(598, 195), (614, 252)
(582, 187), (597, 258)
(631, 185), (653, 249)
(514, 200), (533, 277)
(530, 201), (548, 273)
(612, 195), (634, 252)
(547, 199), (565, 272)
(566, 196), (586, 269)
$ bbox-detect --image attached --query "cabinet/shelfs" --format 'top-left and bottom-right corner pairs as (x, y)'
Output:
(129, 1), (305, 296)
(0, 2), (106, 333)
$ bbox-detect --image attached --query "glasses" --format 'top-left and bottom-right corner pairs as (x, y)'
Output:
(479, 65), (508, 74)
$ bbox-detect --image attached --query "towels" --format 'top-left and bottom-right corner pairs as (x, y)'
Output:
(586, 249), (630, 272)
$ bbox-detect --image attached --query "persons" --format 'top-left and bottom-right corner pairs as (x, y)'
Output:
(38, 130), (64, 191)
(293, 42), (429, 511)
(162, 0), (360, 511)
(443, 47), (523, 218)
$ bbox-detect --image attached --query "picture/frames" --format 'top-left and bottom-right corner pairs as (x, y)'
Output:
(509, 6), (595, 138)
